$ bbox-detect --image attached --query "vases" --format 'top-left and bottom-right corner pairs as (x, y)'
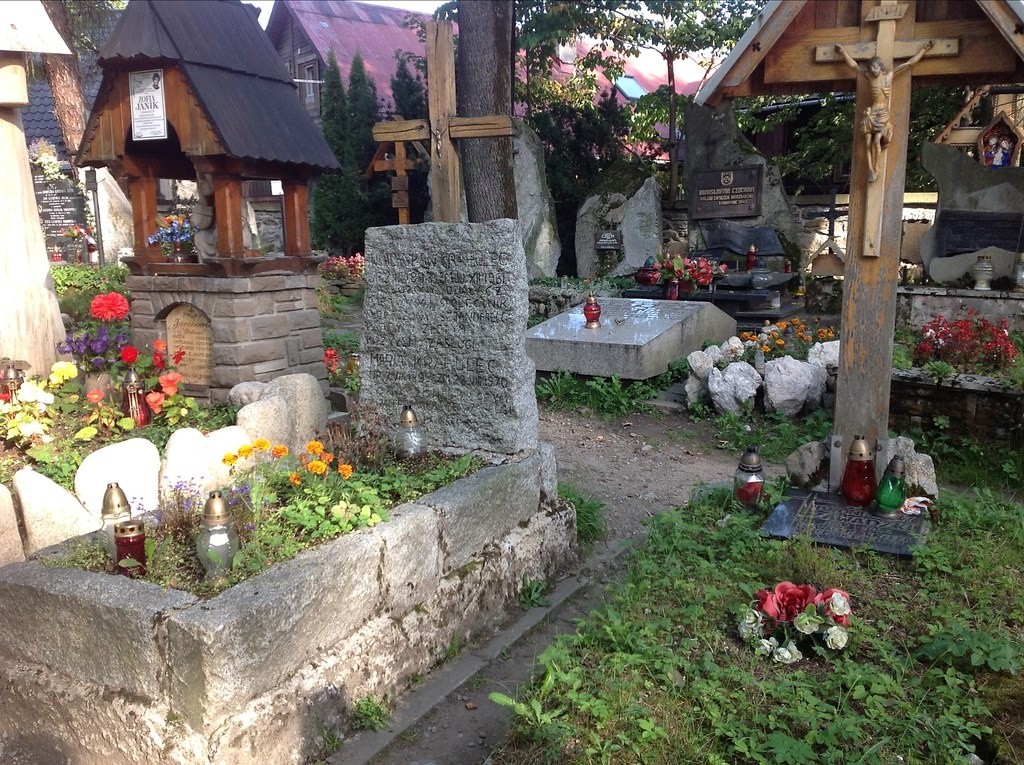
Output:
(168, 240), (191, 263)
(85, 371), (124, 402)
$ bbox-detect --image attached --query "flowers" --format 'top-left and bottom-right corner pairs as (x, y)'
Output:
(148, 212), (200, 244)
(55, 326), (131, 371)
